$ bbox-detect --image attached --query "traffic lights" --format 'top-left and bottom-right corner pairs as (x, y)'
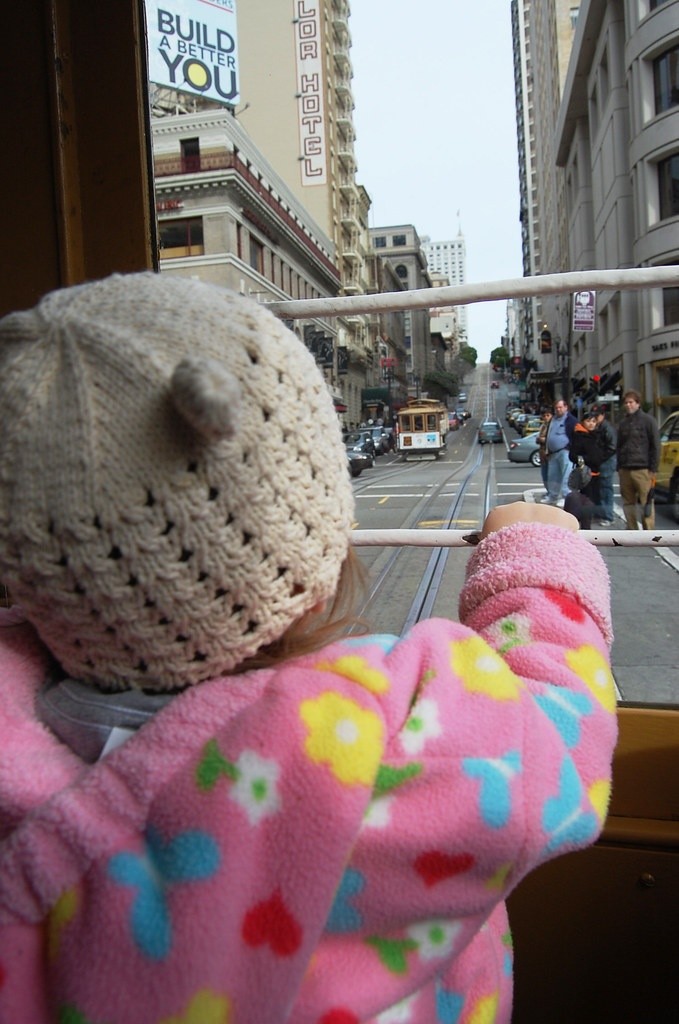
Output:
(541, 331), (554, 354)
(510, 356), (521, 374)
(585, 403), (608, 415)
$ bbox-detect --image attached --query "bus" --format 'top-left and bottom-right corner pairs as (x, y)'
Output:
(395, 399), (450, 456)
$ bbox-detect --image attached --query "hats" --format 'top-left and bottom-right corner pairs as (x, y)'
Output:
(589, 405), (604, 416)
(0, 271), (358, 688)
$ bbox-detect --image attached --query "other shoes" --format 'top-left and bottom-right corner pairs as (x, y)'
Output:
(540, 496), (555, 504)
(556, 499), (565, 507)
(600, 519), (614, 526)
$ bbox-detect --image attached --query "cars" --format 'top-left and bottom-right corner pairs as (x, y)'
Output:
(653, 410), (679, 497)
(448, 392), (472, 431)
(339, 425), (396, 478)
(476, 421), (505, 444)
(490, 380), (502, 389)
(504, 400), (545, 467)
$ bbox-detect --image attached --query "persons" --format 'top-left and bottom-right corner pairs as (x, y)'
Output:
(535, 408), (554, 488)
(594, 408), (616, 526)
(569, 413), (611, 516)
(0, 271), (620, 1023)
(616, 390), (660, 530)
(540, 399), (579, 506)
